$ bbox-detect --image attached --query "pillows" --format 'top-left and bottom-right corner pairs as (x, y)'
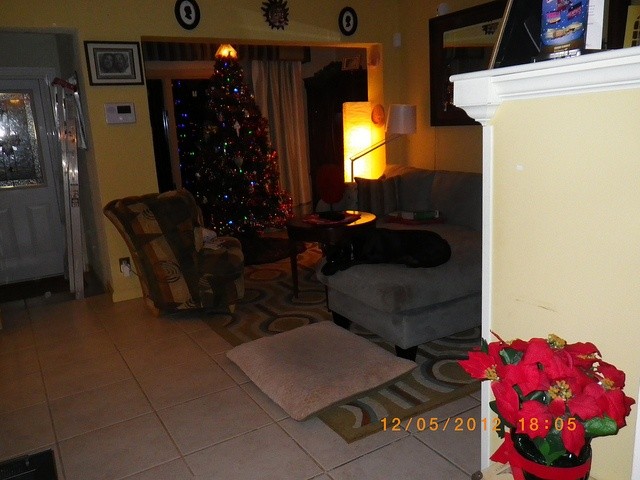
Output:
(354, 176), (401, 217)
(354, 174), (387, 209)
(370, 174), (402, 218)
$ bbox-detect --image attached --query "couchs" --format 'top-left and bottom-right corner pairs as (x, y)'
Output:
(314, 163), (482, 349)
(103, 189), (243, 311)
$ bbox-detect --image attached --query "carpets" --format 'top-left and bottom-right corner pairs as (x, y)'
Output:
(197, 239), (483, 444)
(226, 319), (419, 423)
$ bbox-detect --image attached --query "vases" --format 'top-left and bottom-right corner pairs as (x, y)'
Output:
(497, 433), (593, 480)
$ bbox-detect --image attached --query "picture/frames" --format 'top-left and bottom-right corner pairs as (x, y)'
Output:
(0, 89), (48, 190)
(428, 0), (540, 127)
(174, 1), (201, 31)
(337, 5), (359, 37)
(84, 41), (142, 84)
(341, 53), (360, 69)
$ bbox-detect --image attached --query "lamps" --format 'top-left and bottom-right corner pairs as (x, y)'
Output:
(350, 103), (417, 185)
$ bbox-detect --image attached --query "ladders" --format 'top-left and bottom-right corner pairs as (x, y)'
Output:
(50, 76), (85, 300)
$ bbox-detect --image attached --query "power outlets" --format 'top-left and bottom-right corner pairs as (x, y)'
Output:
(117, 256), (130, 273)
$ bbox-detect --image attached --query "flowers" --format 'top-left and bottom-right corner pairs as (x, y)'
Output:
(457, 328), (636, 464)
